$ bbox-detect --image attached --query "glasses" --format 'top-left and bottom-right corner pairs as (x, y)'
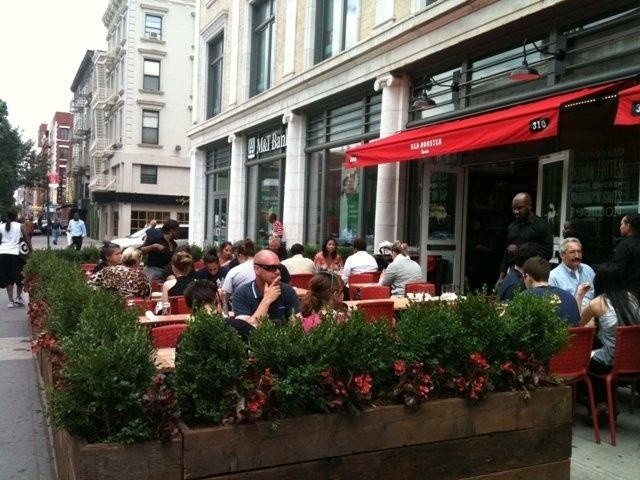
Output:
(320, 270), (333, 291)
(254, 263), (285, 272)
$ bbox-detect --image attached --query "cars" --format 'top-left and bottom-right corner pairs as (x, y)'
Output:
(28, 219), (63, 233)
(110, 222), (189, 249)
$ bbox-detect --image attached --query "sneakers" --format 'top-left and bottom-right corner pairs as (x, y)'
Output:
(584, 401), (609, 424)
(13, 297), (26, 307)
(7, 301), (15, 308)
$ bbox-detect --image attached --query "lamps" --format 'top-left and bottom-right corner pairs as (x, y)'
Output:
(508, 39), (568, 82)
(411, 72), (459, 109)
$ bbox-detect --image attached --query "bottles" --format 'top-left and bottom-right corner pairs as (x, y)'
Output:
(155, 301), (172, 317)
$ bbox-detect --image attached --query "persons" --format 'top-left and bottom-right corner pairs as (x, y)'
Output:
(497, 242), (550, 301)
(547, 237), (596, 309)
(555, 218), (589, 265)
(612, 214), (640, 306)
(314, 236), (424, 299)
(86, 212), (347, 345)
(0, 210), (65, 308)
(470, 217), (495, 294)
(66, 213), (87, 251)
(573, 261), (640, 428)
(494, 191), (551, 291)
(520, 255), (582, 377)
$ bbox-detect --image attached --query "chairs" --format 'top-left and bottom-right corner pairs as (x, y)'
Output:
(546, 326), (601, 444)
(591, 325), (640, 446)
